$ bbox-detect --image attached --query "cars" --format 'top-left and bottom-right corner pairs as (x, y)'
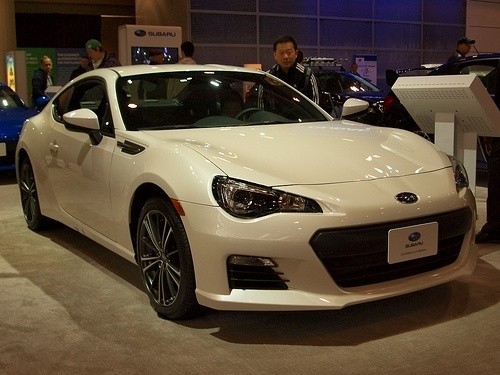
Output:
(314, 70), (395, 129)
(0, 83), (49, 168)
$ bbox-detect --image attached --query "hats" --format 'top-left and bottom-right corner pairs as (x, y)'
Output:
(457, 36), (477, 46)
(146, 48), (165, 58)
(86, 38), (103, 50)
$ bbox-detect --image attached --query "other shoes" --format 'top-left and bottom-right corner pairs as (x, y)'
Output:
(474, 222), (500, 244)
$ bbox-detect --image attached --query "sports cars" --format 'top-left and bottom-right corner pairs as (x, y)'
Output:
(15, 64), (477, 322)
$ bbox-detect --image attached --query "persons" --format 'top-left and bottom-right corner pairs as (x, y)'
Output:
(445, 36), (471, 75)
(256, 36), (321, 120)
(474, 98), (500, 245)
(350, 62), (359, 76)
(31, 36), (197, 112)
